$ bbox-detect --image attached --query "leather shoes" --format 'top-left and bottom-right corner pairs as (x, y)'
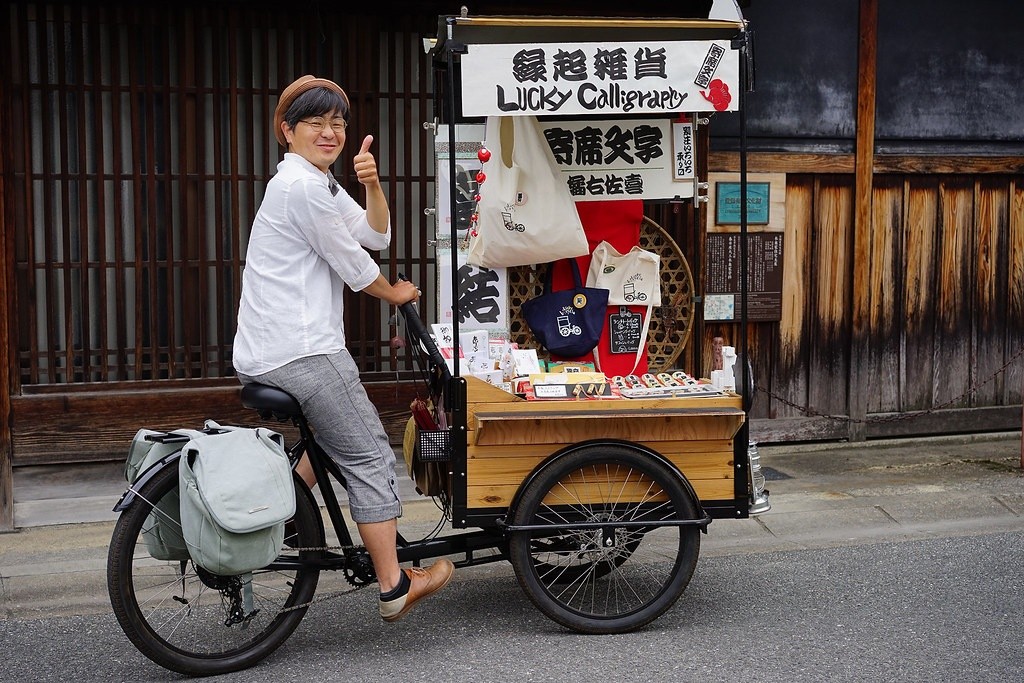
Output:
(379, 559), (454, 622)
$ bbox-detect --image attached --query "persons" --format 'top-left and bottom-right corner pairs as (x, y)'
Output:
(232, 75), (455, 621)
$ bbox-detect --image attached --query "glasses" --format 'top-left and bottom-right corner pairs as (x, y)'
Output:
(300, 116), (347, 133)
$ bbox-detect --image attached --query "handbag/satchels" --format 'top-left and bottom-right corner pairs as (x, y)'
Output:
(585, 241), (661, 379)
(461, 116), (590, 268)
(521, 258), (610, 358)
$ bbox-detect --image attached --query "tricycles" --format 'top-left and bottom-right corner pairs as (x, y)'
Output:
(106, 0), (771, 677)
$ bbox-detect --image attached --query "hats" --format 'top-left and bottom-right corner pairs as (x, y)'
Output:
(274, 75), (350, 147)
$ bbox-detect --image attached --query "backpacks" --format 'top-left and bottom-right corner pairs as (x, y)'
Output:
(124, 418), (296, 631)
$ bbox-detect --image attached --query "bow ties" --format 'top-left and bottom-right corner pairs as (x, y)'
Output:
(328, 170), (340, 197)
